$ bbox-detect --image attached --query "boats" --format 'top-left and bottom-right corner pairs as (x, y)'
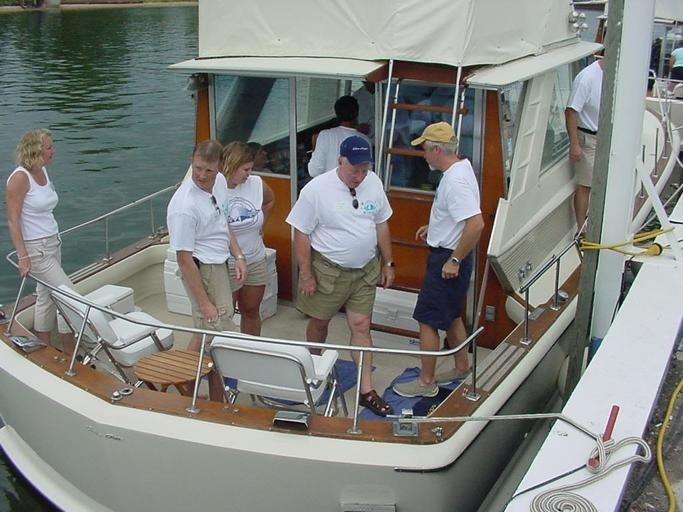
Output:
(0, 1), (681, 512)
(592, 0), (683, 151)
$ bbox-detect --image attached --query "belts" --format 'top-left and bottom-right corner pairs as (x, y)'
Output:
(577, 125), (597, 136)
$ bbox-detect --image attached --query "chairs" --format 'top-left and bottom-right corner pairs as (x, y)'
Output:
(51, 283), (173, 388)
(209, 330), (349, 417)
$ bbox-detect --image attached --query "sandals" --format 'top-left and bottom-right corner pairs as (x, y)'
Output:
(359, 390), (394, 417)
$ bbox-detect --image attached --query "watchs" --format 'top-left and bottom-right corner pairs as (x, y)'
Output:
(384, 262), (394, 267)
(447, 257), (462, 266)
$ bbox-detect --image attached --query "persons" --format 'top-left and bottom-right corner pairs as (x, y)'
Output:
(7, 129), (77, 359)
(393, 121), (484, 399)
(307, 95), (373, 177)
(285, 136), (395, 417)
(167, 141), (248, 403)
(219, 142), (275, 337)
(667, 40), (683, 92)
(354, 81), (407, 145)
(565, 48), (605, 241)
(249, 141), (271, 173)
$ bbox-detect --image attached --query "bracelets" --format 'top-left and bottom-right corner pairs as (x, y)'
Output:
(235, 256), (246, 260)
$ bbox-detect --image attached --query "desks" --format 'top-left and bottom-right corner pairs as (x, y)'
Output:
(134, 350), (214, 395)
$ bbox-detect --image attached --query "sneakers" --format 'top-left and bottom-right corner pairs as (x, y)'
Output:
(436, 367), (473, 385)
(392, 379), (439, 398)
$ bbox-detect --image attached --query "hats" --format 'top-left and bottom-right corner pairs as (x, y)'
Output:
(339, 136), (375, 167)
(411, 121), (459, 146)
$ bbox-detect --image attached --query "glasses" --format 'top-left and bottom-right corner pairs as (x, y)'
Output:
(211, 194), (221, 215)
(349, 187), (359, 210)
